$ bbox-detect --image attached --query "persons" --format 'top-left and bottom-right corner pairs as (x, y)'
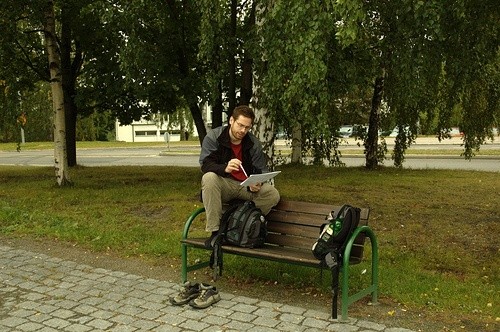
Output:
(199, 104), (280, 250)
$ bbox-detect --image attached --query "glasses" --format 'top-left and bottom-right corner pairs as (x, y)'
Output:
(236, 122), (250, 128)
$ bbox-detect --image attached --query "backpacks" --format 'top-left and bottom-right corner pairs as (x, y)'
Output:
(311, 205), (362, 264)
(220, 200), (268, 249)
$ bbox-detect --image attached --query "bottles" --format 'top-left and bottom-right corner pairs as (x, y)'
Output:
(311, 214), (345, 256)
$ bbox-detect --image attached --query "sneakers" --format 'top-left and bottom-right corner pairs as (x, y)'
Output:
(168, 281), (200, 305)
(190, 283), (220, 308)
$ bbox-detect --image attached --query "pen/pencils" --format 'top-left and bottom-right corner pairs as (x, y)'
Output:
(239, 164), (250, 177)
